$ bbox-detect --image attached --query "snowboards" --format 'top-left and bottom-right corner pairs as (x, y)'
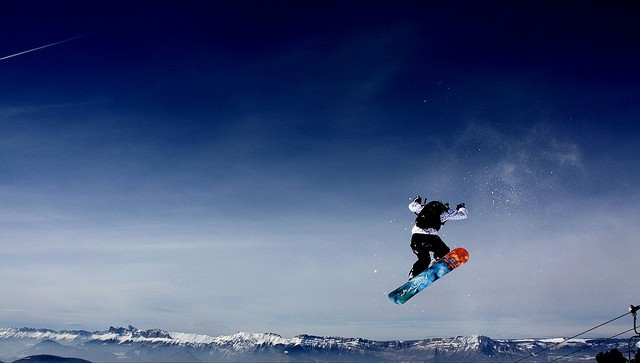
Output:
(387, 247), (470, 305)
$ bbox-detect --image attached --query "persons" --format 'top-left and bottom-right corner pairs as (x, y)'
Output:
(406, 195), (468, 280)
